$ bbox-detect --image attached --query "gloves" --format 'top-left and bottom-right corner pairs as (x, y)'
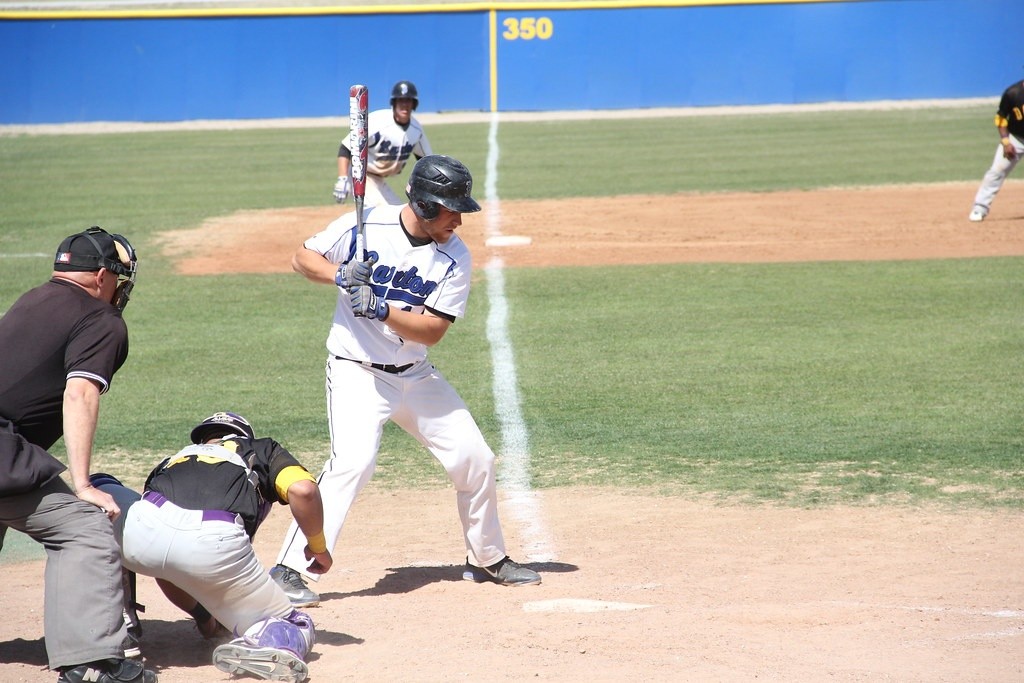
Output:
(344, 285), (390, 321)
(332, 176), (352, 205)
(334, 260), (373, 288)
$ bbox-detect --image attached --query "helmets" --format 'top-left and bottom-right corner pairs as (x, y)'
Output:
(404, 155), (481, 219)
(190, 411), (254, 441)
(389, 80), (419, 111)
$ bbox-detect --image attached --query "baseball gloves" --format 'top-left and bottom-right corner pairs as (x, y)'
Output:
(192, 603), (231, 642)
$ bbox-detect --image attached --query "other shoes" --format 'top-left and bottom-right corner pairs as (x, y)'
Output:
(969, 203), (989, 222)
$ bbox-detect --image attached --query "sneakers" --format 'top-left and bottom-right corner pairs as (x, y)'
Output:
(269, 566), (320, 607)
(121, 620), (142, 658)
(57, 658), (158, 683)
(212, 643), (308, 683)
(463, 555), (542, 586)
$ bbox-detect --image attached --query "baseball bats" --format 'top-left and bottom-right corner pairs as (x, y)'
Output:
(349, 85), (369, 317)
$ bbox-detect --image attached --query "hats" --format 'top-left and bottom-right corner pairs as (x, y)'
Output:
(55, 225), (131, 277)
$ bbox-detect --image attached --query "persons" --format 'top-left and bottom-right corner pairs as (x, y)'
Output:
(270, 154), (541, 609)
(968, 79), (1024, 222)
(0, 225), (160, 683)
(89, 412), (333, 683)
(333, 81), (433, 208)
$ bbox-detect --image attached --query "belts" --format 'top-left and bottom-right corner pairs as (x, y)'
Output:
(336, 355), (414, 373)
(142, 490), (238, 523)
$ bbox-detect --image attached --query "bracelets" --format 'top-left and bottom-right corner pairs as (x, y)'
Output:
(305, 530), (326, 553)
(1001, 138), (1009, 146)
